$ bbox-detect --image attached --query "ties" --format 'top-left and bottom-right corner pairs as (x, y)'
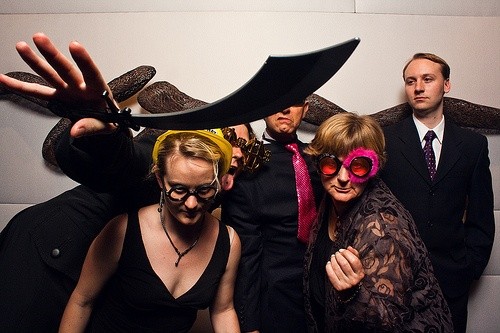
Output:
(287, 143), (316, 244)
(423, 131), (436, 181)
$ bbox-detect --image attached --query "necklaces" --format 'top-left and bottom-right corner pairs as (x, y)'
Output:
(158, 204), (205, 266)
(327, 196), (351, 236)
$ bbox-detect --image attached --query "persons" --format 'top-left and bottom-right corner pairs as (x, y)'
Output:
(303, 111), (456, 333)
(54, 116), (243, 333)
(224, 94), (341, 333)
(362, 51), (494, 333)
(1, 30), (254, 333)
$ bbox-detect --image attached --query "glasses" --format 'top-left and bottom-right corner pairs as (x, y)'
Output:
(318, 147), (379, 184)
(161, 178), (217, 203)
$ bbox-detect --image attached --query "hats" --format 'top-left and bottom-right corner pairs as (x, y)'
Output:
(152, 130), (232, 177)
